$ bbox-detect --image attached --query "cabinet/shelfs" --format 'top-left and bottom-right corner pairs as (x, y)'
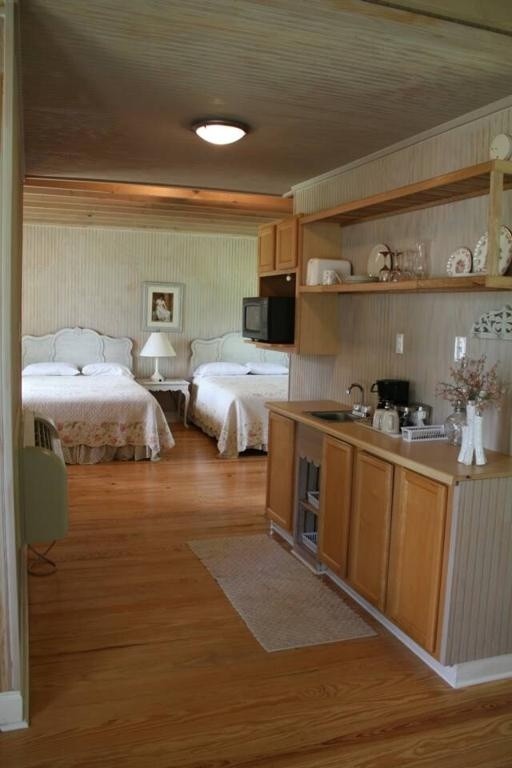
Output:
(264, 409), (297, 534)
(295, 158), (511, 294)
(316, 433), (396, 614)
(384, 462), (449, 659)
(256, 215), (298, 278)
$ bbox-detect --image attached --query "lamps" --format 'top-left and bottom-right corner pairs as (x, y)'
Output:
(138, 331), (178, 382)
(189, 117), (253, 146)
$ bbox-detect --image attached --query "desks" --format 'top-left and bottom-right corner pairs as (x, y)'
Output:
(133, 378), (192, 431)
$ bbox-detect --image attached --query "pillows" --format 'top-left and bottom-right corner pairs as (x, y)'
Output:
(246, 361), (289, 376)
(81, 361), (136, 377)
(193, 360), (254, 376)
(22, 361), (81, 377)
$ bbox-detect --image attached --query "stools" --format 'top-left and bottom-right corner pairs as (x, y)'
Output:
(19, 445), (68, 578)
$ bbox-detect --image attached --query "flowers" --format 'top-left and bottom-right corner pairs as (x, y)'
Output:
(433, 350), (512, 417)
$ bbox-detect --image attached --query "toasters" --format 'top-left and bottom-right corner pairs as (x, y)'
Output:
(306, 257), (352, 286)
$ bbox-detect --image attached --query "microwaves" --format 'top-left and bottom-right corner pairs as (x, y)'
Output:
(240, 295), (295, 342)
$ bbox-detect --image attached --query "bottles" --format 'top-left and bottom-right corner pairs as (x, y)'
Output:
(404, 401), (431, 425)
(443, 406), (465, 448)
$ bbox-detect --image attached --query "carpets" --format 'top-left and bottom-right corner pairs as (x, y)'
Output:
(185, 532), (380, 663)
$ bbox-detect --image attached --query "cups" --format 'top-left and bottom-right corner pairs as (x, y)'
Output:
(383, 411), (399, 433)
(321, 269), (336, 285)
(372, 408), (386, 430)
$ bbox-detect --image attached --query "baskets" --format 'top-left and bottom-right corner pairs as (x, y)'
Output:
(302, 532), (316, 552)
(402, 424), (447, 442)
(307, 491), (319, 510)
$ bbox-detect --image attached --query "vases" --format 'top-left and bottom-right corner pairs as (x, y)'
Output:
(456, 400), (490, 467)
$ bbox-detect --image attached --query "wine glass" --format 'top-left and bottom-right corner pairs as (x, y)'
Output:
(378, 240), (427, 281)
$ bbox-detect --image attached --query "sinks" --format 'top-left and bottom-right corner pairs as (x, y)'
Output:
(304, 410), (356, 423)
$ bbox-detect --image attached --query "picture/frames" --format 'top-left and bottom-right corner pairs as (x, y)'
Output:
(141, 280), (184, 333)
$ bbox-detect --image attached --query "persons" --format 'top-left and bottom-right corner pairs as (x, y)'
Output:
(155, 295), (171, 322)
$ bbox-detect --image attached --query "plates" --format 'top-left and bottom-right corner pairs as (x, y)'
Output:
(342, 275), (377, 282)
(471, 225), (511, 276)
(366, 243), (391, 279)
(446, 248), (470, 276)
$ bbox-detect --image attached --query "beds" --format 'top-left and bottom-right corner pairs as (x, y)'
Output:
(186, 332), (289, 460)
(20, 328), (177, 466)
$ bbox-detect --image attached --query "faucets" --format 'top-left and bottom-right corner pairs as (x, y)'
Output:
(346, 384), (365, 406)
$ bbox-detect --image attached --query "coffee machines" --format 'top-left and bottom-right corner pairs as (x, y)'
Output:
(375, 378), (409, 434)
